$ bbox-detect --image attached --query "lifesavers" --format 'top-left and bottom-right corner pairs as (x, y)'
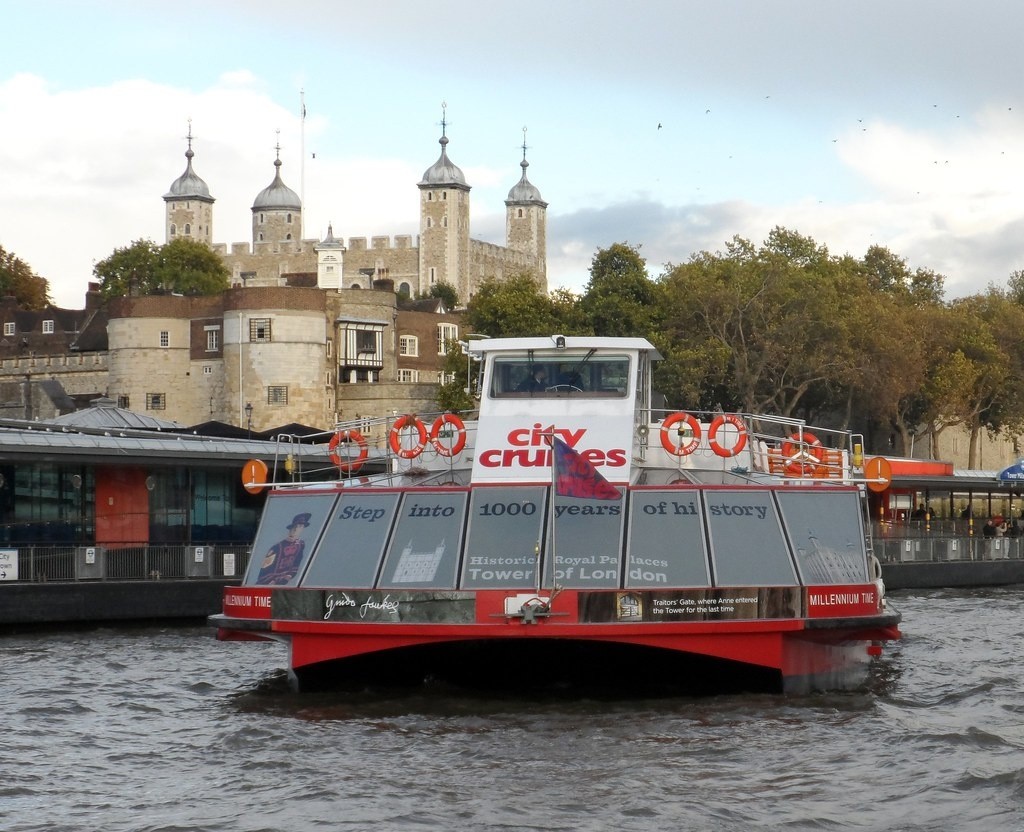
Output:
(708, 415), (746, 457)
(329, 430), (368, 472)
(782, 432), (823, 475)
(389, 416), (428, 459)
(430, 413), (467, 457)
(660, 412), (701, 456)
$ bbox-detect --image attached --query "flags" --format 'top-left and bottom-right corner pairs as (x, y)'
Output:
(553, 435), (624, 501)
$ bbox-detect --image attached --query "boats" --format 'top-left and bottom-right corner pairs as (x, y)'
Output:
(854, 452), (1024, 591)
(0, 393), (400, 635)
(200, 331), (903, 706)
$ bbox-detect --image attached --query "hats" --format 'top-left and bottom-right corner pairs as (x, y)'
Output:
(286, 513), (311, 529)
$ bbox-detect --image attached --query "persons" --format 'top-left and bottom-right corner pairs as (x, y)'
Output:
(514, 363), (551, 393)
(915, 504), (927, 519)
(983, 519), (1024, 539)
(252, 513), (312, 587)
(962, 505), (974, 517)
(558, 364), (584, 392)
(928, 507), (936, 520)
